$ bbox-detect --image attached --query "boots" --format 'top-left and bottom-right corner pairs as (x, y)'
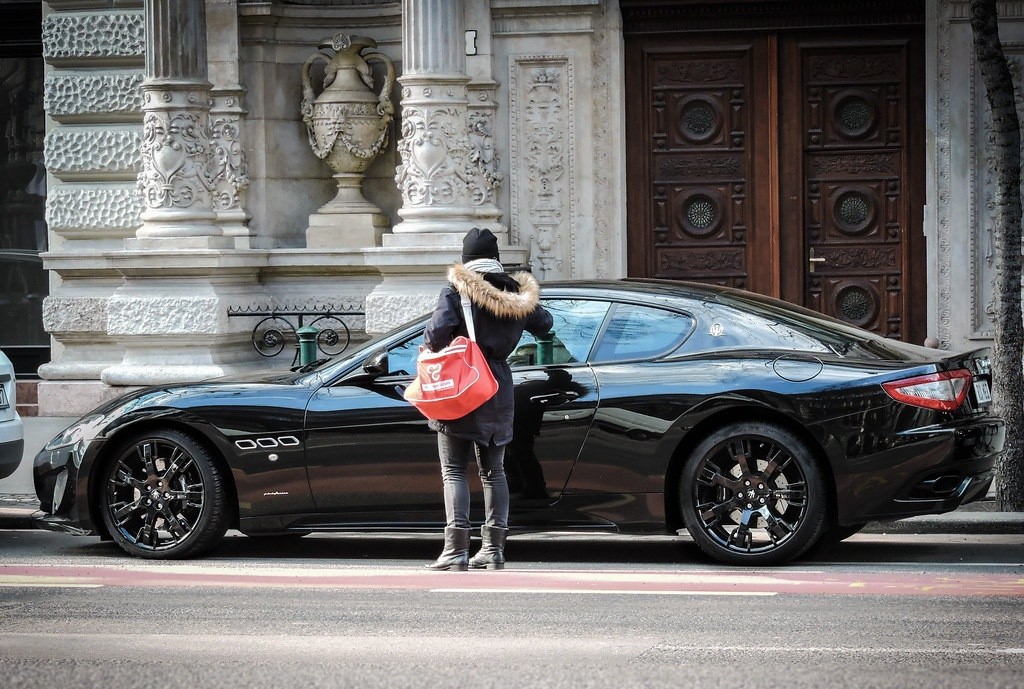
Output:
(424, 526), (473, 571)
(468, 525), (509, 569)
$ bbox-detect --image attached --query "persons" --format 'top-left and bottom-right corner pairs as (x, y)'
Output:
(504, 343), (574, 499)
(423, 227), (554, 572)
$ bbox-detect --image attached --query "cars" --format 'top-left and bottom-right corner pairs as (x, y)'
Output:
(0, 350), (25, 479)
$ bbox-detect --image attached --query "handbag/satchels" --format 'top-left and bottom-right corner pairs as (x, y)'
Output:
(403, 291), (499, 420)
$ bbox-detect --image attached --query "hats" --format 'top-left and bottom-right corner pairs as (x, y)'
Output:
(462, 227), (500, 263)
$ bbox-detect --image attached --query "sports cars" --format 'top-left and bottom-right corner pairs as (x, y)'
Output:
(34, 277), (1015, 566)
